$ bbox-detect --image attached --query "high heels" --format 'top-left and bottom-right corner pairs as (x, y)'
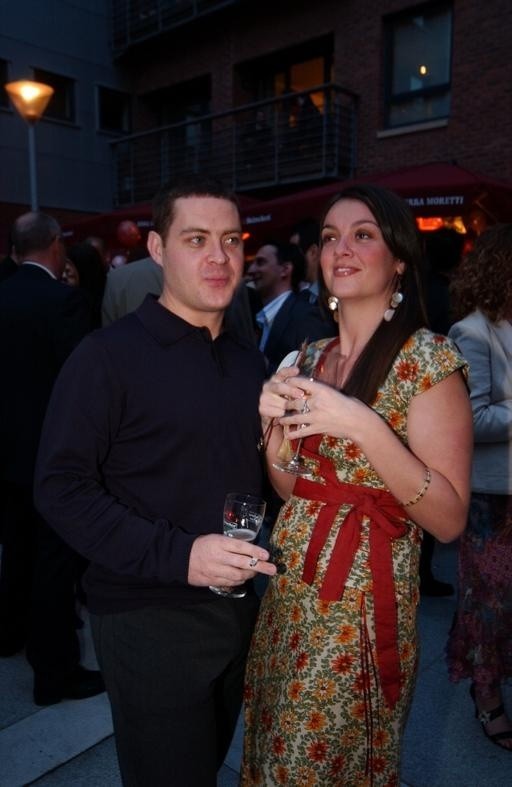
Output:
(469, 684), (512, 752)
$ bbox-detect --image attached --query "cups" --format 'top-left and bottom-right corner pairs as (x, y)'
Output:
(208, 492), (267, 541)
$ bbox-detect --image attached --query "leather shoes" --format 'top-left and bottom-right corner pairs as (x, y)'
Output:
(28, 661), (109, 708)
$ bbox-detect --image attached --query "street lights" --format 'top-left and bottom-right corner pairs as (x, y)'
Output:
(5, 78), (54, 213)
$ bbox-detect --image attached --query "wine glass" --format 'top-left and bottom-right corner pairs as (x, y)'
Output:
(271, 345), (347, 477)
